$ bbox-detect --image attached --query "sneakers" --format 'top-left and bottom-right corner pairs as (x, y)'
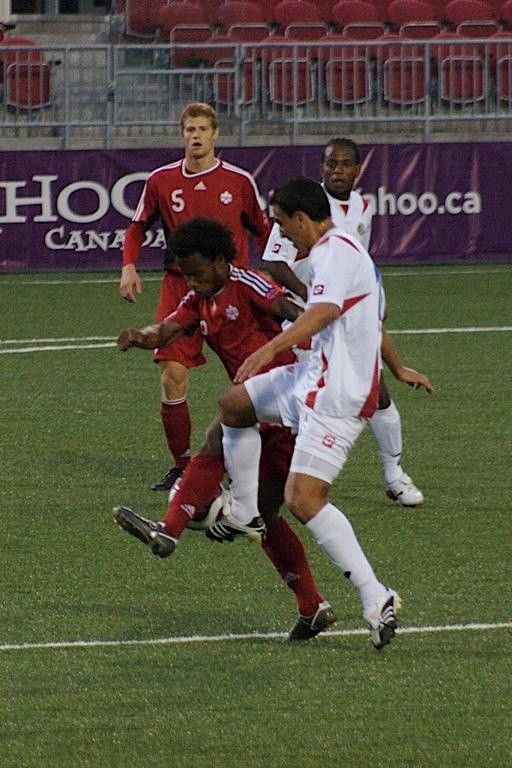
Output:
(283, 601), (337, 641)
(363, 587), (403, 649)
(151, 468), (184, 491)
(113, 499), (268, 558)
(384, 473), (424, 505)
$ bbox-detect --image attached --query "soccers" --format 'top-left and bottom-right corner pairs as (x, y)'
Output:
(169, 478), (227, 530)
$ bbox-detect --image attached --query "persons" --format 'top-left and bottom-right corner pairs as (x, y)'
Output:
(110, 218), (337, 642)
(117, 100), (273, 491)
(262, 138), (425, 508)
(207, 175), (434, 649)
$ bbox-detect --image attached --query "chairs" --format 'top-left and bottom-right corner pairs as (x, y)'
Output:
(111, 0), (512, 107)
(0, 37), (43, 82)
(6, 62), (51, 110)
